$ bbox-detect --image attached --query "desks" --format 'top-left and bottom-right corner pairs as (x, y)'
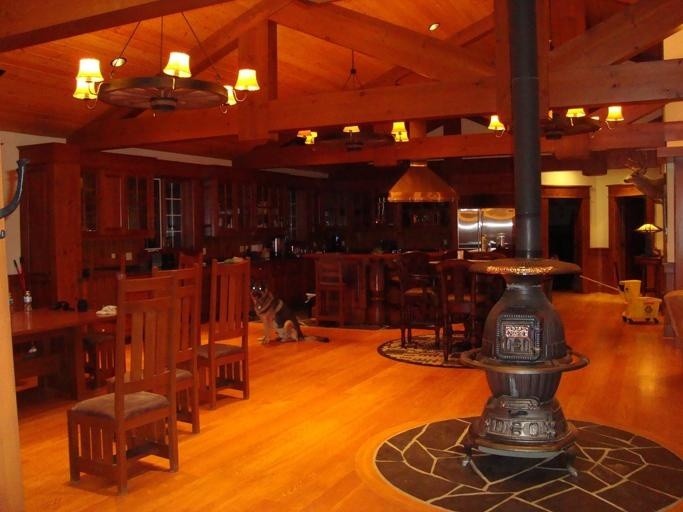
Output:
(10, 298), (90, 406)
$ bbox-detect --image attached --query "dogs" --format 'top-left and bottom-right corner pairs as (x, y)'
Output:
(250, 272), (329, 344)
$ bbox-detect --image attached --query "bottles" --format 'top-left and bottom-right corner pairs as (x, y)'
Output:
(23, 290), (33, 312)
(9, 293), (14, 313)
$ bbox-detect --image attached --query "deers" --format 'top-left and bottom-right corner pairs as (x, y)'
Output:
(623, 151), (666, 204)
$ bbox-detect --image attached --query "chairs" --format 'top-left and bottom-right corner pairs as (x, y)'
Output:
(68, 269), (182, 498)
(16, 140), (517, 305)
(300, 250), (484, 361)
(105, 264), (204, 437)
(175, 251), (251, 409)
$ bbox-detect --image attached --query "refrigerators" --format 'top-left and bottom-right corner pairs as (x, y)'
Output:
(456, 208), (515, 253)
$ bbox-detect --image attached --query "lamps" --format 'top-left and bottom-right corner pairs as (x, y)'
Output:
(486, 26), (625, 140)
(635, 219), (661, 258)
(66, 3), (259, 121)
(292, 46), (410, 149)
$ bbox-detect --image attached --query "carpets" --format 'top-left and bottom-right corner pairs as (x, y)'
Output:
(375, 331), (474, 368)
